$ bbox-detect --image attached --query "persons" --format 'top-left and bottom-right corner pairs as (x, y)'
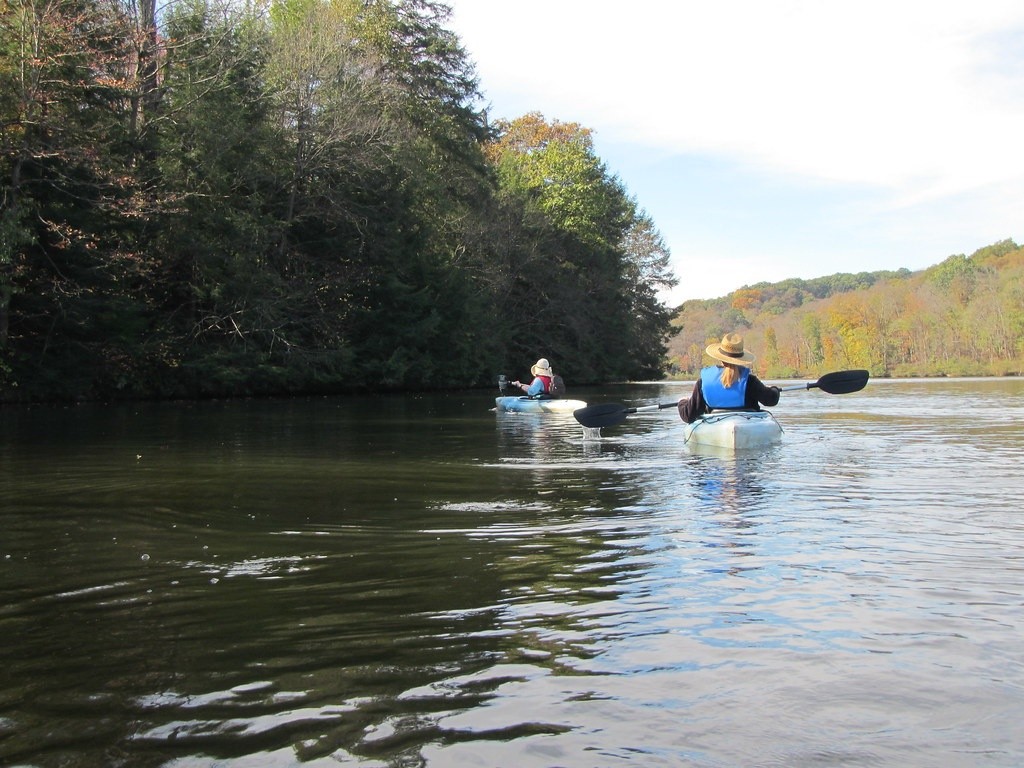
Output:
(677, 333), (780, 423)
(508, 358), (561, 400)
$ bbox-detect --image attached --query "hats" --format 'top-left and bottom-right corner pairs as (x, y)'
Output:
(531, 358), (554, 377)
(705, 332), (756, 365)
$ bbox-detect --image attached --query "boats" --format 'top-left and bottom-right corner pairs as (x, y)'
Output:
(685, 412), (784, 451)
(495, 397), (588, 413)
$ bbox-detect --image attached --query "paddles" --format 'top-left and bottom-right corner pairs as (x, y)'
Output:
(497, 375), (536, 396)
(574, 369), (869, 428)
